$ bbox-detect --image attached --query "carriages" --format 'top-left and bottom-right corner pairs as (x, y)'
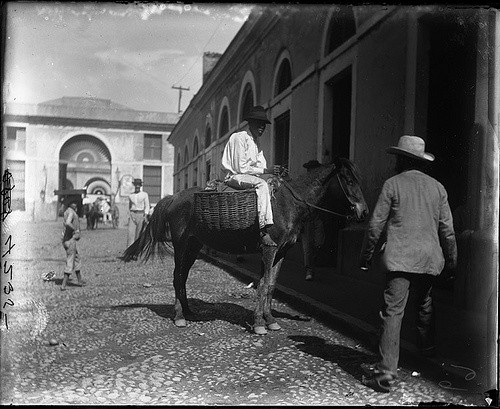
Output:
(85, 193), (119, 229)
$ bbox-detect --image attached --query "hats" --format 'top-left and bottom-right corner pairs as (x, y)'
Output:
(244, 106), (271, 124)
(132, 179), (143, 184)
(386, 135), (435, 162)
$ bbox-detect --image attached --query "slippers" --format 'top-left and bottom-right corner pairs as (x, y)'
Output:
(361, 373), (390, 392)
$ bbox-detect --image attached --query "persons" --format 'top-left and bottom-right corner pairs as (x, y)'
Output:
(220, 106), (290, 247)
(301, 159), (320, 280)
(126, 179), (150, 262)
(360, 136), (458, 383)
(60, 198), (87, 290)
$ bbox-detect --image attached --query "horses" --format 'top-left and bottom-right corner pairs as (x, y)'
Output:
(120, 157), (369, 333)
